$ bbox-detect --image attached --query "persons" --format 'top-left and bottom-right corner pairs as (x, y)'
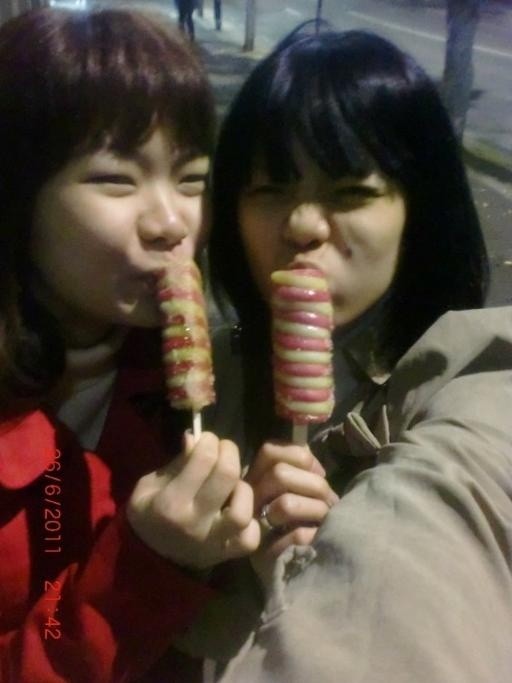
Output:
(174, 0), (196, 41)
(0, 5), (265, 679)
(172, 15), (511, 681)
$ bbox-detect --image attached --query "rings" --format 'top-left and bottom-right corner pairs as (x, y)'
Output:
(260, 503), (275, 530)
